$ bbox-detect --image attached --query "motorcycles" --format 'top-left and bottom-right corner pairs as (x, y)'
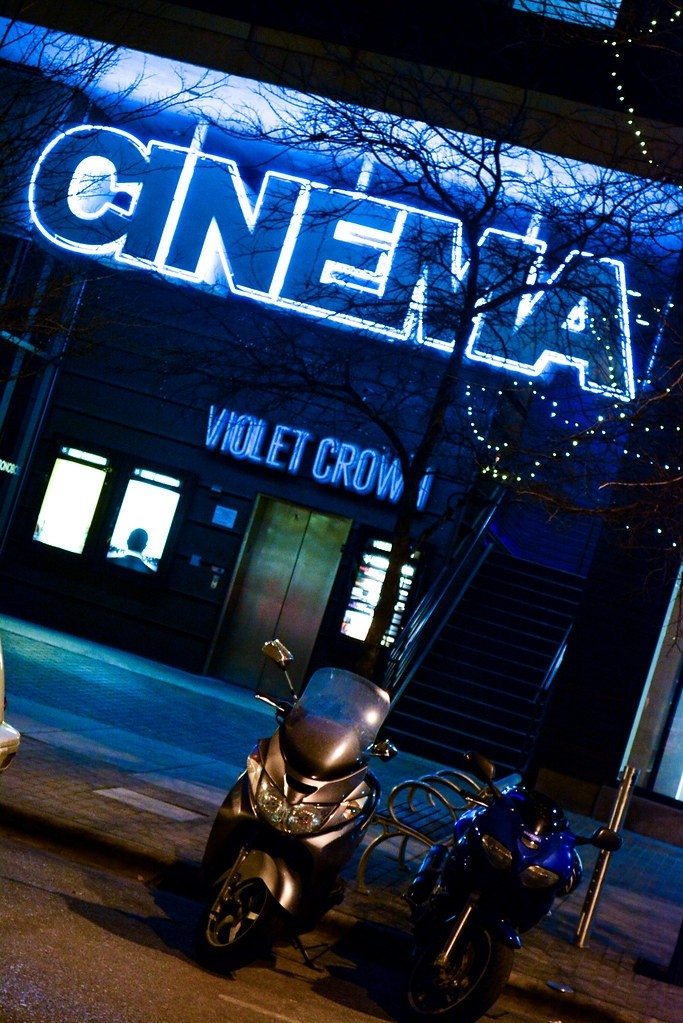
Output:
(384, 750), (625, 1022)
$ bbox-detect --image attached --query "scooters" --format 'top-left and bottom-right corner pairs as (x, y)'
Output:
(193, 640), (405, 977)
